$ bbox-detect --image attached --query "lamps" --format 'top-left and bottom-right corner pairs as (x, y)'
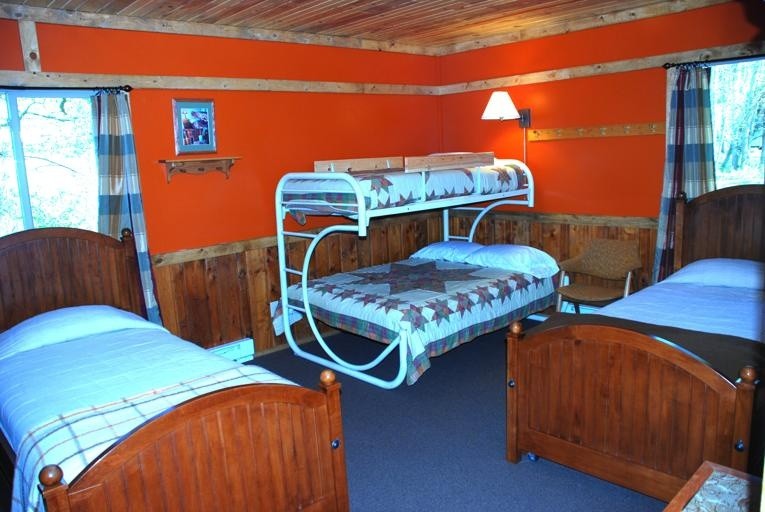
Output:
(480, 90), (531, 128)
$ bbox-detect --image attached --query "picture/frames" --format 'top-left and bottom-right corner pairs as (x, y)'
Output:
(171, 98), (217, 156)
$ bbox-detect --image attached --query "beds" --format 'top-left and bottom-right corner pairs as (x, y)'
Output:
(504, 184), (763, 502)
(274, 153), (560, 390)
(0, 227), (349, 512)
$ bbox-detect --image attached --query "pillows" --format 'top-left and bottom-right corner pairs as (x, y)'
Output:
(408, 240), (560, 280)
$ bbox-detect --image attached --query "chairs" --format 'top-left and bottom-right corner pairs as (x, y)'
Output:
(557, 235), (643, 317)
